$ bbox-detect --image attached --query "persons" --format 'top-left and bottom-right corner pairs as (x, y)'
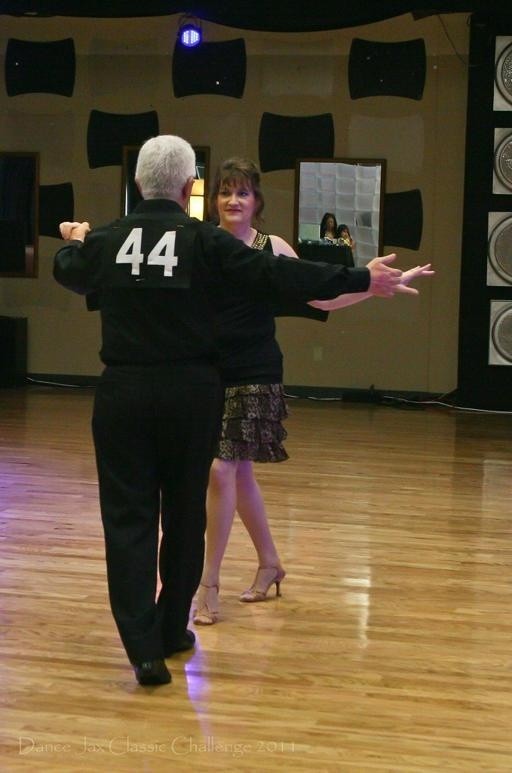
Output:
(58, 157), (435, 627)
(337, 223), (355, 249)
(53, 133), (403, 685)
(320, 212), (337, 239)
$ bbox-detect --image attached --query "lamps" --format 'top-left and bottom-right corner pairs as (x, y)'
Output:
(178, 15), (202, 47)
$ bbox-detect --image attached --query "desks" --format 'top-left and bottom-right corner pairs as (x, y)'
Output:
(299, 240), (354, 268)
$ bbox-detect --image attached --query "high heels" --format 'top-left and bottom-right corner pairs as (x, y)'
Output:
(240, 567), (285, 602)
(193, 583), (220, 625)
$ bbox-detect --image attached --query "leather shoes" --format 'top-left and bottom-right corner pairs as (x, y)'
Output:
(134, 657), (171, 685)
(163, 630), (195, 658)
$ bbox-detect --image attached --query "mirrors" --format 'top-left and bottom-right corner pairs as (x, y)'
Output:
(120, 144), (211, 225)
(0, 150), (39, 279)
(292, 157), (387, 268)
